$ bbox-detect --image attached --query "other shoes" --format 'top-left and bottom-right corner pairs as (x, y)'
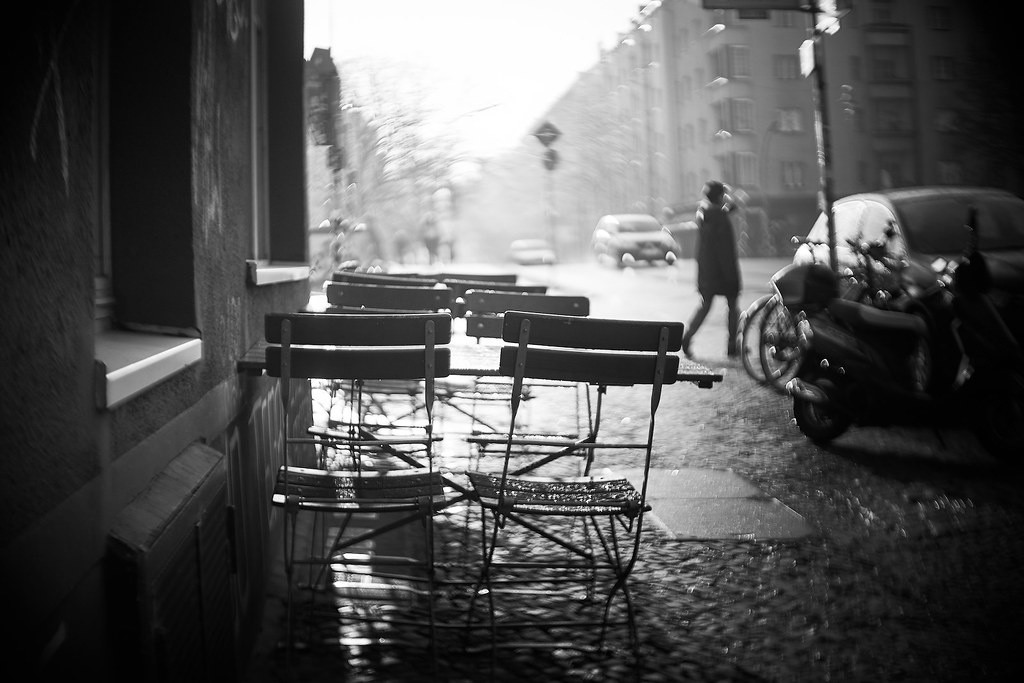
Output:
(681, 339), (690, 355)
(728, 347), (749, 358)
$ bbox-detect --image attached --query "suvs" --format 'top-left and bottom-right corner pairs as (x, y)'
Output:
(791, 183), (1023, 344)
(593, 212), (681, 268)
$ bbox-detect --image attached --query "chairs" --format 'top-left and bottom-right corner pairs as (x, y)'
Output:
(258, 266), (685, 649)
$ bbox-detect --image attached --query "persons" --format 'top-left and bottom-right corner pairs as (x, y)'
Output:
(682, 179), (751, 356)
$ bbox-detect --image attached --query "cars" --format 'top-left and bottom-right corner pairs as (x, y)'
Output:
(512, 238), (553, 264)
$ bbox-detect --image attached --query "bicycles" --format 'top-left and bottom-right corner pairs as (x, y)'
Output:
(740, 231), (831, 389)
(760, 216), (904, 399)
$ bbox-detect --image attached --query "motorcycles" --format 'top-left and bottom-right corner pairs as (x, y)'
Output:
(768, 200), (1024, 472)
